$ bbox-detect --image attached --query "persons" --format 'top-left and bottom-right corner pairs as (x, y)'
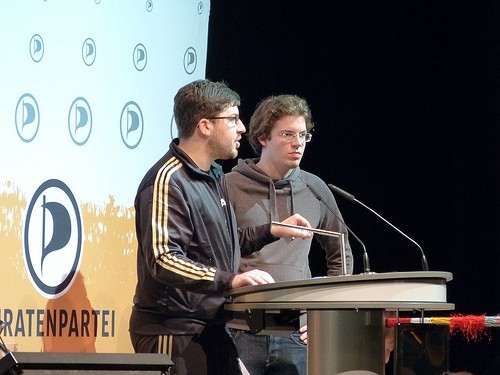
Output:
(383, 310), (476, 375)
(224, 95), (354, 375)
(129, 79), (313, 375)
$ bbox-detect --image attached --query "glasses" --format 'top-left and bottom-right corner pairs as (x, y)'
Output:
(197, 115), (239, 128)
(264, 131), (312, 142)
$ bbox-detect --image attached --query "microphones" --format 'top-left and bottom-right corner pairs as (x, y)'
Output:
(328, 183), (428, 273)
(306, 183), (370, 275)
